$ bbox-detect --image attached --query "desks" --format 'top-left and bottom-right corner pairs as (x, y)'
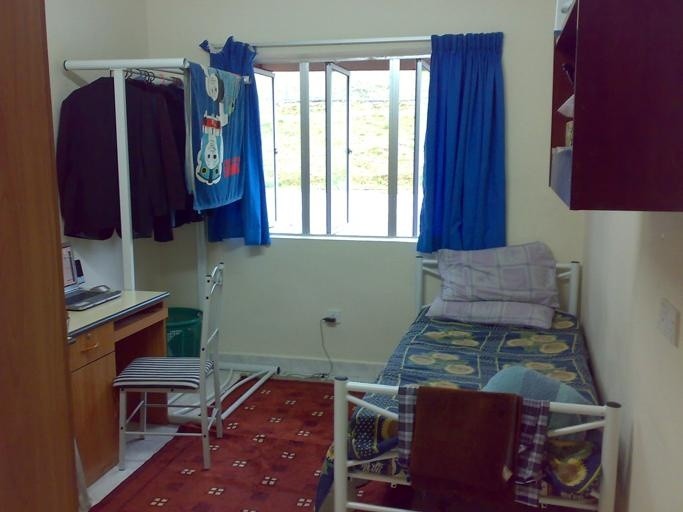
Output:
(60, 291), (173, 483)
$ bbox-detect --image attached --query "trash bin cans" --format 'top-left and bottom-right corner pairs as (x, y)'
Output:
(165, 306), (203, 357)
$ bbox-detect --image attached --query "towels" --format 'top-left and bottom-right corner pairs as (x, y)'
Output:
(190, 60), (247, 211)
(409, 383), (522, 510)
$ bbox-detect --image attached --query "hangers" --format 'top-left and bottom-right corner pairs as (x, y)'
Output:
(63, 66), (185, 110)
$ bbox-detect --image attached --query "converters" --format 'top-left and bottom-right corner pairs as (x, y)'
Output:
(323, 317), (336, 323)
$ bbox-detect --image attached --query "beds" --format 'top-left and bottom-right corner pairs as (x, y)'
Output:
(317, 256), (622, 511)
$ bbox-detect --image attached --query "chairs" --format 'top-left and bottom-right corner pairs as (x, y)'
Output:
(111, 262), (225, 471)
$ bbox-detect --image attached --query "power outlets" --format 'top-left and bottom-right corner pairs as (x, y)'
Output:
(323, 311), (341, 326)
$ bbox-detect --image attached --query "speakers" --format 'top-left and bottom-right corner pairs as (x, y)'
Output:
(74, 259), (83, 284)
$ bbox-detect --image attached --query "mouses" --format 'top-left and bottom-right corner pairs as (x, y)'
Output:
(90, 285), (110, 293)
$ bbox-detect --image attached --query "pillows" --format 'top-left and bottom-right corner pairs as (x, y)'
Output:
(440, 242), (557, 307)
(426, 293), (552, 331)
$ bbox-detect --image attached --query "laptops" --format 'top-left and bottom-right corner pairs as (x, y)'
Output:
(61, 242), (122, 311)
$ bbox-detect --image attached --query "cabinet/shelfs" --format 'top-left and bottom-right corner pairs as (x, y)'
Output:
(545, 0), (683, 213)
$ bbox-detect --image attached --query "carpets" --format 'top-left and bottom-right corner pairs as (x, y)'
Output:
(87, 377), (365, 512)
